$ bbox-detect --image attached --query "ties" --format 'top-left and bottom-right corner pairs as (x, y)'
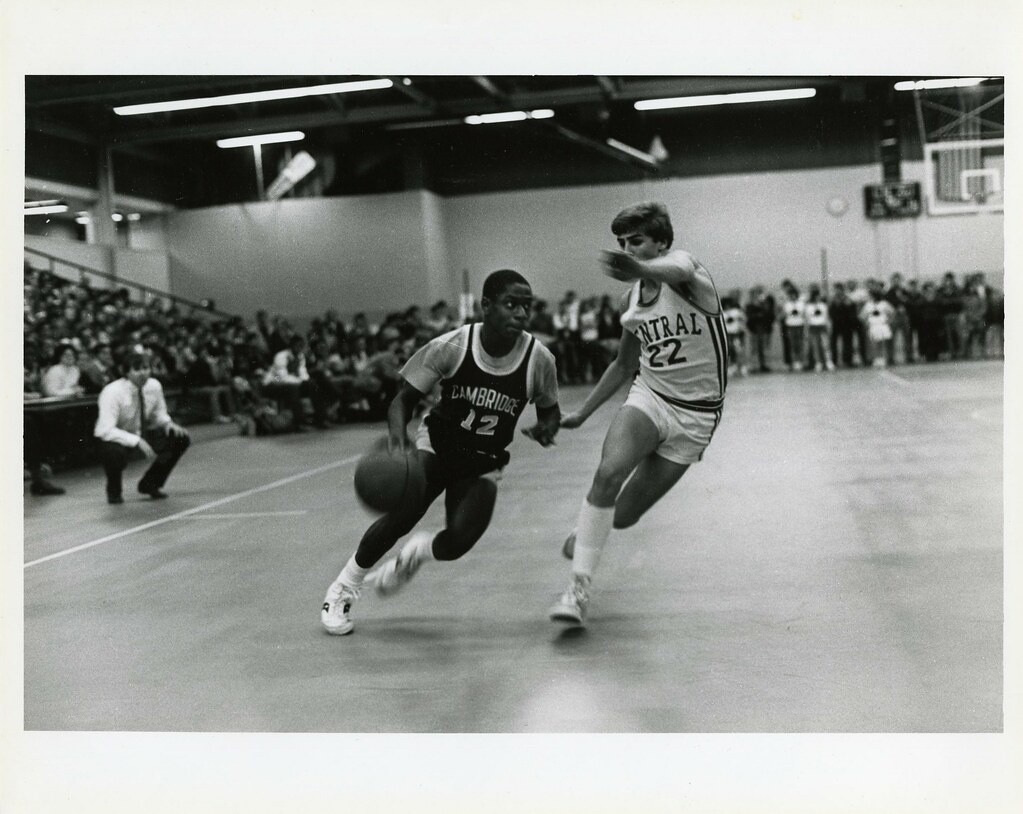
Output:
(140, 388), (146, 439)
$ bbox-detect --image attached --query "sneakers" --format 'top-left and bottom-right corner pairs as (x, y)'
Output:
(320, 581), (356, 635)
(376, 529), (429, 598)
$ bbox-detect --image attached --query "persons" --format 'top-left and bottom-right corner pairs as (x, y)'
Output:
(322, 268), (562, 633)
(550, 199), (731, 627)
(93, 353), (192, 505)
(24, 265), (1004, 500)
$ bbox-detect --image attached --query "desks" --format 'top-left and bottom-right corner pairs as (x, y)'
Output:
(24, 397), (103, 470)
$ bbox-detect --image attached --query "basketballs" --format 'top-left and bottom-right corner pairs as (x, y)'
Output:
(352, 445), (426, 517)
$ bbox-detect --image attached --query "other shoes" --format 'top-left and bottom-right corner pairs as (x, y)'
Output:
(30, 481), (66, 495)
(564, 530), (578, 559)
(551, 576), (589, 624)
(139, 481), (168, 500)
(109, 491), (125, 504)
